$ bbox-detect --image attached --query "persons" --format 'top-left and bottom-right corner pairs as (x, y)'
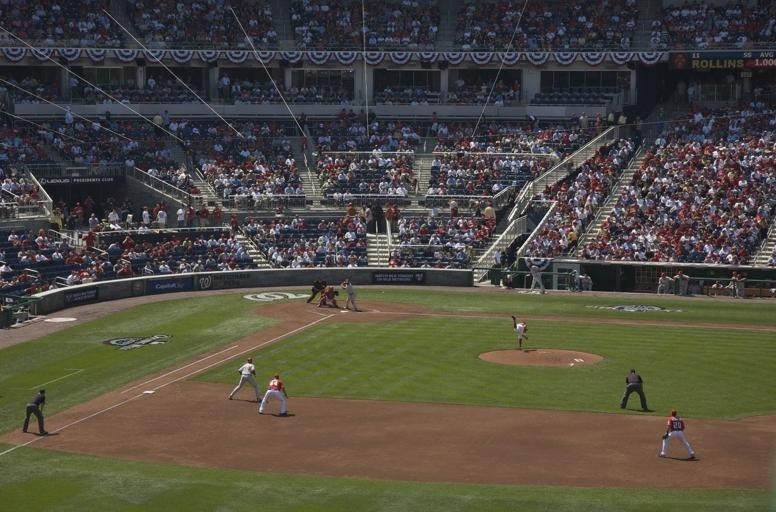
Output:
(228, 357), (262, 403)
(22, 389), (49, 436)
(259, 373), (289, 416)
(511, 316), (528, 347)
(659, 409), (695, 459)
(620, 368), (649, 411)
